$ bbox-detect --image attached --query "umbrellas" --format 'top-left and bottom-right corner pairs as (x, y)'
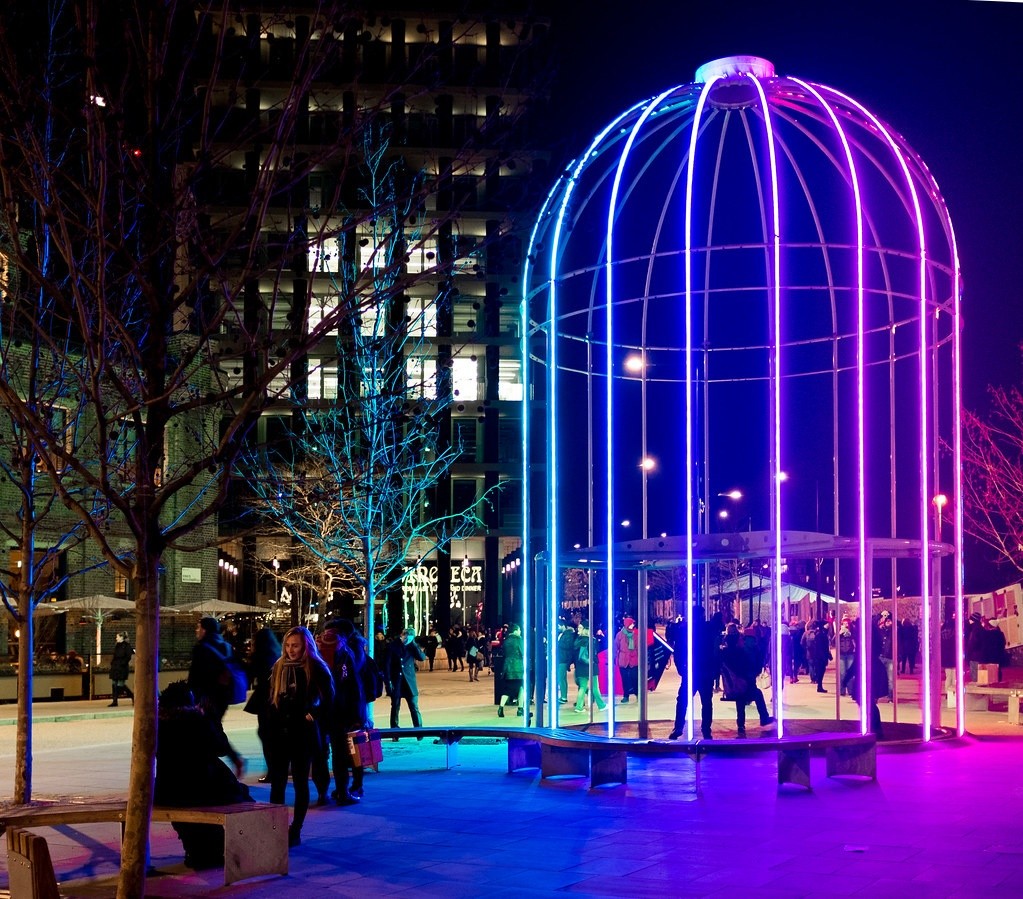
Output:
(174, 599), (275, 620)
(49, 594), (192, 667)
(0, 595), (69, 618)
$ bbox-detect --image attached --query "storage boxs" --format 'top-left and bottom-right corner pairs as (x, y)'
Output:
(977, 663), (1000, 685)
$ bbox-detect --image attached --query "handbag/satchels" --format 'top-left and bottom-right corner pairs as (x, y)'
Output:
(347, 728), (383, 767)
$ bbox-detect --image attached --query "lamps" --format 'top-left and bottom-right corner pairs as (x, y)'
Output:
(511, 542), (516, 568)
(516, 538), (521, 565)
(416, 542), (421, 567)
(501, 547), (505, 574)
(230, 545), (235, 573)
(233, 546), (239, 575)
(506, 546), (510, 571)
(461, 538), (469, 567)
(225, 543), (229, 570)
(219, 544), (224, 567)
(272, 539), (279, 568)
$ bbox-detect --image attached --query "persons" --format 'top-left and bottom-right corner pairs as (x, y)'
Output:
(615, 618), (638, 703)
(423, 630), (439, 672)
(189, 616), (248, 778)
(156, 679), (256, 864)
(443, 626), (508, 682)
(497, 624), (533, 717)
(557, 619), (608, 712)
(108, 631), (134, 707)
(67, 650), (83, 671)
(375, 627), (425, 742)
(665, 605), (919, 740)
(942, 609), (1007, 693)
(317, 620), (373, 806)
(260, 626), (336, 848)
(243, 628), (282, 782)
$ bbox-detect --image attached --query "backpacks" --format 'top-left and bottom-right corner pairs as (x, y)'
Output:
(206, 642), (248, 705)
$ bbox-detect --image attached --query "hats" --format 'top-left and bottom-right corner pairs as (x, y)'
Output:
(789, 621), (798, 627)
(969, 612), (981, 622)
(842, 614), (850, 623)
(989, 620), (1000, 628)
(198, 617), (220, 632)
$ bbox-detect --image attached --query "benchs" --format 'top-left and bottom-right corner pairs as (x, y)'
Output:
(0, 788), (292, 886)
(946, 682), (1023, 726)
(357, 720), (883, 799)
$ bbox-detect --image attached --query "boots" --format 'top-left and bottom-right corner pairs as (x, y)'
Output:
(108, 685), (118, 706)
(120, 685), (133, 698)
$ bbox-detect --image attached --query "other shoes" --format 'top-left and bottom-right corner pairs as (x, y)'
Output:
(574, 709), (585, 713)
(498, 706), (504, 718)
(349, 785), (364, 795)
(599, 704), (607, 712)
(621, 695), (629, 703)
(517, 707), (524, 716)
(702, 732), (713, 739)
(336, 791), (361, 806)
(817, 688), (827, 692)
(288, 825), (301, 845)
(318, 793), (329, 804)
(235, 757), (247, 779)
(760, 717), (773, 726)
(669, 729), (683, 739)
(558, 698), (568, 704)
(258, 775), (271, 783)
(736, 733), (746, 739)
